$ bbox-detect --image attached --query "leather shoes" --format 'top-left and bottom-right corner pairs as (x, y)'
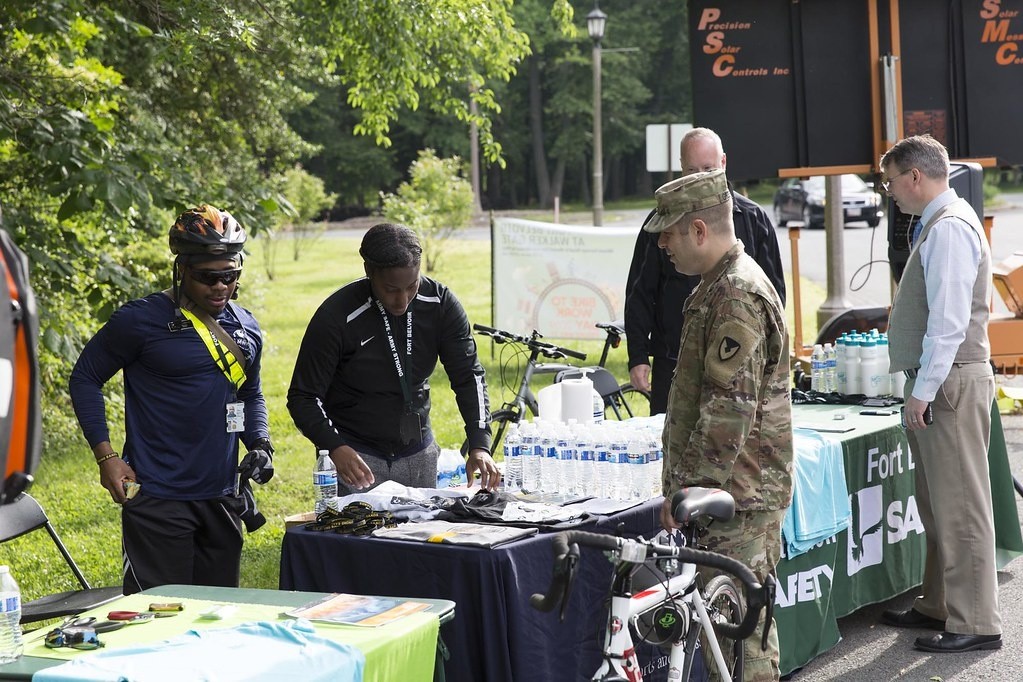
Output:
(882, 606), (946, 631)
(914, 632), (1002, 653)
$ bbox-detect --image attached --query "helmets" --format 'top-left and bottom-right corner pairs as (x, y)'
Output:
(168, 204), (248, 257)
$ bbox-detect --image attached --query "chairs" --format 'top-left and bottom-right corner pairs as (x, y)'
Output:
(0, 491), (123, 624)
(554, 367), (634, 421)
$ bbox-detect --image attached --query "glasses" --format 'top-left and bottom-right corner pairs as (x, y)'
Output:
(185, 260), (242, 286)
(881, 169), (912, 192)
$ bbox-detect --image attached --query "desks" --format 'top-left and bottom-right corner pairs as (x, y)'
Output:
(0, 396), (1023, 682)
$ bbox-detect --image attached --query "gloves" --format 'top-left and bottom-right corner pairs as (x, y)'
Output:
(240, 449), (274, 485)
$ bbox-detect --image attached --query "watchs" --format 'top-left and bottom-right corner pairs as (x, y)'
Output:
(251, 439), (275, 451)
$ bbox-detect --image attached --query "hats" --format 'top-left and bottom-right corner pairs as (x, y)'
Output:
(641, 169), (732, 233)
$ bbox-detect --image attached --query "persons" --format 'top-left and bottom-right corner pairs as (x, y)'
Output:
(623, 128), (785, 419)
(68, 203), (275, 597)
(287, 223), (503, 500)
(645, 169), (795, 682)
(875, 135), (1003, 653)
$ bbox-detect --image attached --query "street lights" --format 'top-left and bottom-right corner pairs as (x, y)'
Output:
(585, 3), (609, 229)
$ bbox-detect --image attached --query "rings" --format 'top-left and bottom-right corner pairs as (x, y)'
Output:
(913, 420), (918, 423)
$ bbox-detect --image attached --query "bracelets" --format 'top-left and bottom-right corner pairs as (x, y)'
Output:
(97, 452), (118, 465)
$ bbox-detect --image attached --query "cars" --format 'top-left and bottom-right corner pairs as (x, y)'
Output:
(774, 171), (878, 232)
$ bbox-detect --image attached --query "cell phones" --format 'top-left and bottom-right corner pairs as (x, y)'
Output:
(901, 402), (933, 427)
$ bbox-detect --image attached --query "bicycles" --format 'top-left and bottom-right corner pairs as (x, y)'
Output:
(524, 483), (775, 681)
(460, 321), (656, 464)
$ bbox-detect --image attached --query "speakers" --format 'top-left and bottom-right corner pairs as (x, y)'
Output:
(888, 161), (984, 284)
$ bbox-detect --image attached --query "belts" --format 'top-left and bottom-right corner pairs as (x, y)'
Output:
(903, 367), (921, 379)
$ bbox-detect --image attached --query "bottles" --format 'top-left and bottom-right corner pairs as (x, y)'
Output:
(312, 450), (339, 520)
(0, 565), (24, 665)
(811, 328), (909, 400)
(505, 414), (672, 504)
